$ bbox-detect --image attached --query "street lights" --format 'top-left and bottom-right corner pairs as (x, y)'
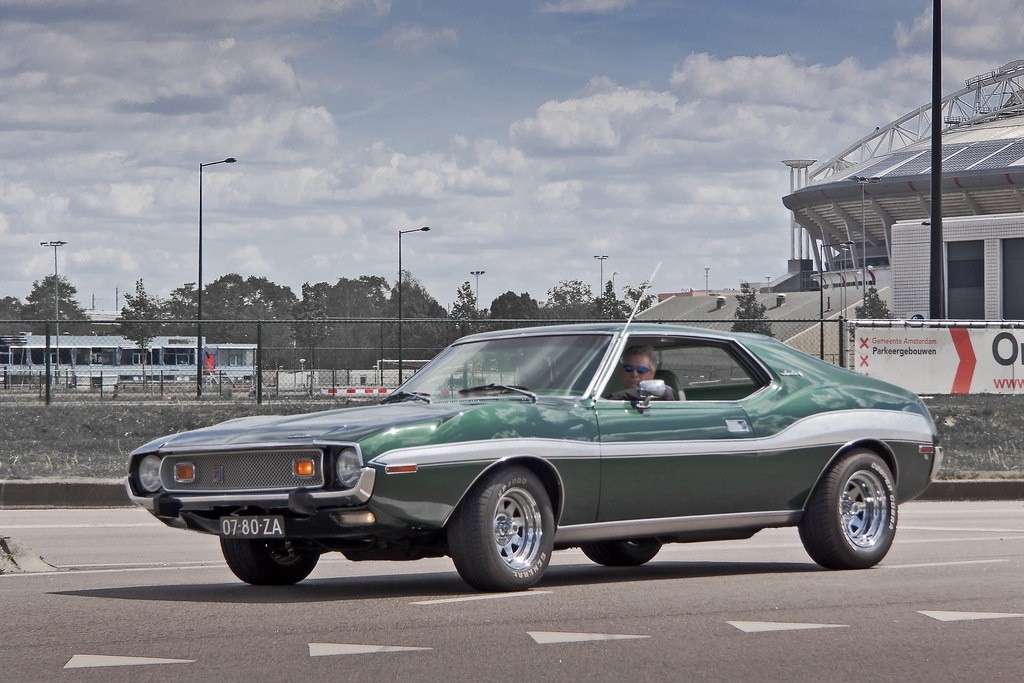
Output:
(398, 226), (431, 387)
(594, 255), (609, 301)
(41, 240), (69, 376)
(197, 157), (237, 394)
(471, 271), (486, 312)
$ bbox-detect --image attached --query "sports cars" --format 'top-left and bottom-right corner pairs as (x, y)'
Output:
(123, 320), (945, 592)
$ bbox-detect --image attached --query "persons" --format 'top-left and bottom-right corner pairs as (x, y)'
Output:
(611, 344), (676, 401)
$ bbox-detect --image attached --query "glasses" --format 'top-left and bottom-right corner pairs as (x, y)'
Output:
(623, 364), (652, 374)
